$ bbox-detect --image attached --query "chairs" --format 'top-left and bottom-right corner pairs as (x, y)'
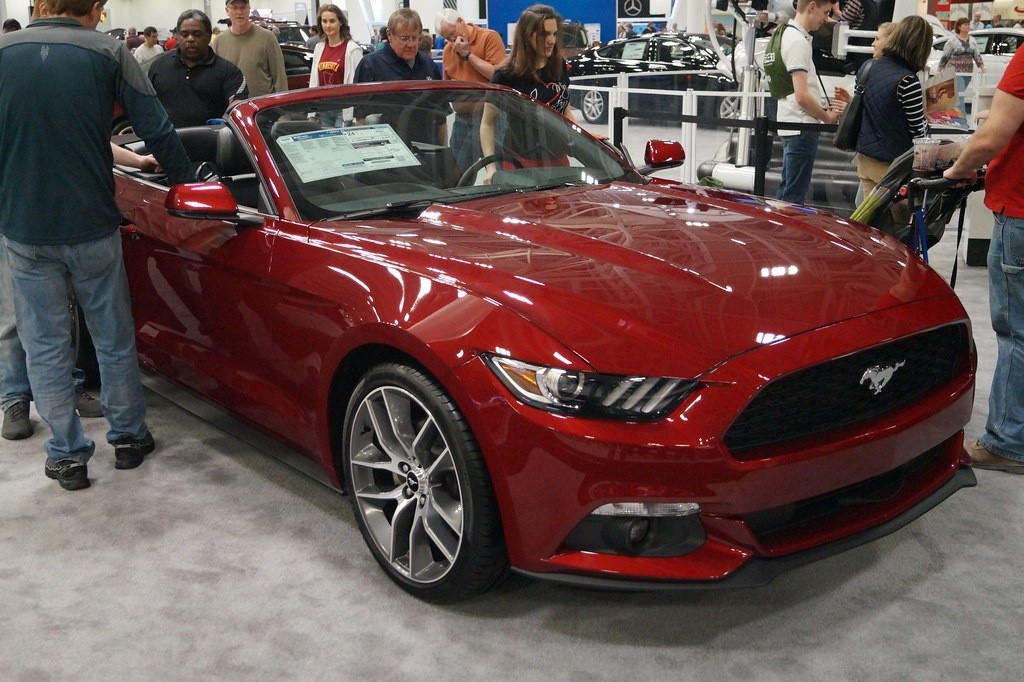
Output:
(174, 126), (260, 207)
(272, 120), (347, 196)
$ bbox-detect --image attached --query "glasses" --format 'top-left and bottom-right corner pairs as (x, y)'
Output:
(393, 32), (419, 44)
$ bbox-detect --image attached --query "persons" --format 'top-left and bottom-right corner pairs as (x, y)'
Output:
(309, 4), (364, 129)
(134, 26), (164, 65)
(821, 0), (864, 60)
(969, 12), (1024, 53)
(939, 16), (987, 120)
(146, 9), (250, 127)
(716, 10), (780, 121)
(773, 0), (841, 206)
(352, 9), (455, 172)
(944, 42), (1024, 477)
(0, 0), (210, 490)
(837, 16), (933, 240)
(211, 0), (288, 99)
(435, 7), (506, 179)
(307, 25), (321, 50)
(642, 23), (657, 35)
(617, 24), (637, 39)
(125, 26), (145, 51)
(662, 27), (667, 32)
(210, 27), (221, 41)
(165, 28), (180, 50)
(478, 5), (609, 187)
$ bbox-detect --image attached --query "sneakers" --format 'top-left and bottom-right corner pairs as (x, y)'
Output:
(44, 457), (91, 491)
(114, 430), (155, 469)
(3, 401), (34, 441)
(963, 440), (1024, 473)
(75, 387), (105, 418)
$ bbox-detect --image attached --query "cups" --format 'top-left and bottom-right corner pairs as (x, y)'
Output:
(912, 138), (940, 170)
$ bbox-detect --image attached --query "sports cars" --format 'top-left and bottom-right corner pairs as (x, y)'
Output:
(68, 78), (984, 603)
(563, 33), (742, 132)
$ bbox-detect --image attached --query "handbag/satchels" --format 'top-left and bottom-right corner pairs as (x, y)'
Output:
(832, 59), (878, 152)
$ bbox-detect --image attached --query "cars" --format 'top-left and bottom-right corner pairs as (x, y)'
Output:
(716, 35), (860, 116)
(915, 27), (1024, 119)
(255, 15), (375, 91)
